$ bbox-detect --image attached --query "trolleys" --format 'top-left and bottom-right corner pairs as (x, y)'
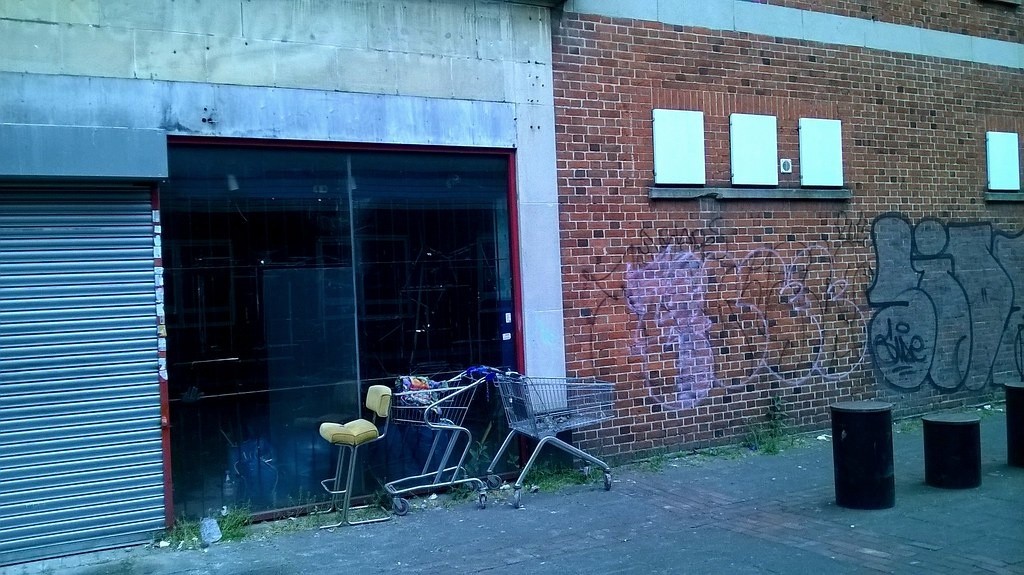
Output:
(384, 366), (496, 515)
(487, 367), (615, 509)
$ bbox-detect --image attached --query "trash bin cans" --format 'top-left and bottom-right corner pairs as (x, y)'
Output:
(828, 401), (893, 510)
(920, 415), (983, 488)
(1003, 383), (1024, 469)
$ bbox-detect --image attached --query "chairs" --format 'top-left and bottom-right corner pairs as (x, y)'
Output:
(316, 385), (391, 530)
